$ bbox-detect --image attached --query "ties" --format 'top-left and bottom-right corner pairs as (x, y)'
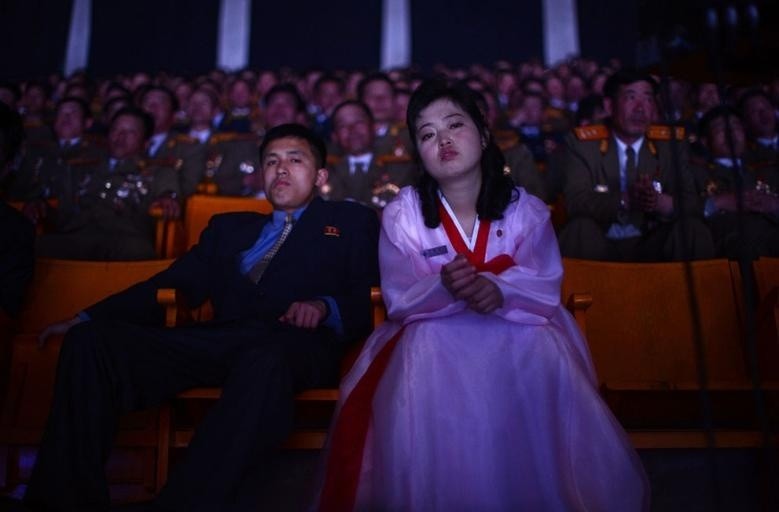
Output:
(354, 162), (365, 200)
(248, 214), (293, 285)
(626, 146), (635, 191)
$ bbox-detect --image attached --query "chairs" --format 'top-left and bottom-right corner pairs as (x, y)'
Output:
(2, 193), (778, 504)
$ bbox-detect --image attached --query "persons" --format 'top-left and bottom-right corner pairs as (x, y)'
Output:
(351, 72), (654, 510)
(20, 121), (380, 512)
(0, 100), (40, 392)
(1, 52), (779, 262)
